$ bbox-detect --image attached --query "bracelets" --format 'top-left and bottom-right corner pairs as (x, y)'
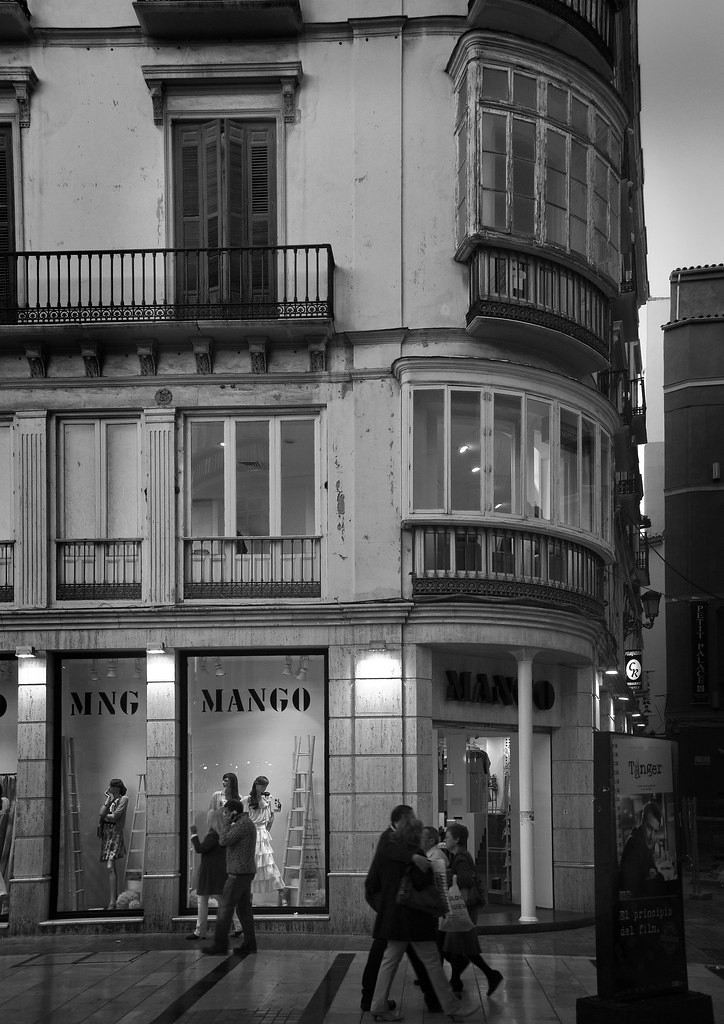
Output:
(103, 803), (108, 806)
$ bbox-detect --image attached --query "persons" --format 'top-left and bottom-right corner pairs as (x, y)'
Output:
(240, 776), (286, 894)
(201, 799), (257, 955)
(620, 803), (665, 892)
(185, 809), (243, 940)
(209, 772), (243, 811)
(99, 779), (129, 909)
(360, 804), (504, 1024)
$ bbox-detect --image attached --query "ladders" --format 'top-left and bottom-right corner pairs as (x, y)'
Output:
(120, 773), (146, 909)
(61, 736), (87, 911)
(278, 734), (325, 908)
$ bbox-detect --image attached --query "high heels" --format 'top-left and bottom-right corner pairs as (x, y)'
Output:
(371, 1011), (404, 1021)
(448, 1004), (483, 1022)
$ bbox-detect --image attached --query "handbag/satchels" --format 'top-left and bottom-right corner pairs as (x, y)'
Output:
(438, 877), (476, 934)
(96, 824), (102, 838)
(396, 868), (448, 915)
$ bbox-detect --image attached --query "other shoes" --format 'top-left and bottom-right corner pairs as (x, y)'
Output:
(450, 979), (464, 992)
(233, 942), (258, 954)
(426, 993), (463, 1011)
(185, 934), (206, 939)
(360, 999), (397, 1012)
(231, 931), (242, 938)
(200, 945), (228, 955)
(486, 970), (503, 996)
